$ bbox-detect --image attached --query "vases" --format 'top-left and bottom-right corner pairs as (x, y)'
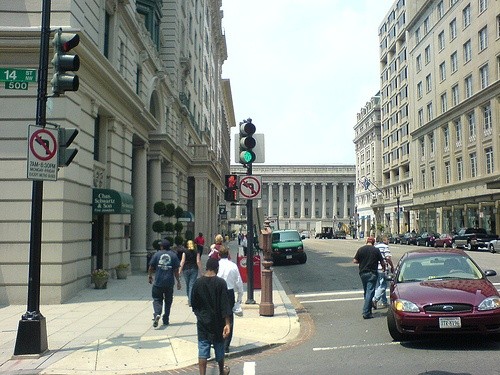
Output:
(92, 279), (109, 289)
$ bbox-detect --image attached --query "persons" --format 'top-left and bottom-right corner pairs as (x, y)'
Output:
(190, 259), (234, 375)
(208, 231), (248, 266)
(179, 239), (204, 307)
(439, 259), (456, 277)
(404, 227), (459, 239)
(148, 239), (182, 327)
(194, 232), (205, 260)
(394, 232), (399, 244)
(210, 247), (244, 354)
(370, 235), (395, 310)
(353, 237), (386, 319)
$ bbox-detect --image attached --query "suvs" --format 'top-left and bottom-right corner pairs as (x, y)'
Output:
(272, 229), (307, 265)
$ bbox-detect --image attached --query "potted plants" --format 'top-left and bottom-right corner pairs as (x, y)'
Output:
(115, 263), (128, 279)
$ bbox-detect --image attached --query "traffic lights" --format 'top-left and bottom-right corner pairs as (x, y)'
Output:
(222, 175), (238, 200)
(55, 128), (78, 166)
(51, 27), (80, 96)
(238, 118), (257, 165)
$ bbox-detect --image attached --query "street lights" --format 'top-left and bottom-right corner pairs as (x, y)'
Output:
(395, 195), (401, 234)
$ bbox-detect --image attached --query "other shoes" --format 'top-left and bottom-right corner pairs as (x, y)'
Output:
(371, 302), (377, 310)
(210, 344), (229, 353)
(153, 313), (161, 327)
(363, 314), (373, 319)
(163, 321), (169, 325)
(220, 365), (230, 375)
(382, 303), (391, 306)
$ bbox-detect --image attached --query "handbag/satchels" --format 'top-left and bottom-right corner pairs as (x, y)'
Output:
(232, 302), (243, 316)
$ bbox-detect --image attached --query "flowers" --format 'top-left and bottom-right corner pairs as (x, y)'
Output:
(92, 269), (110, 279)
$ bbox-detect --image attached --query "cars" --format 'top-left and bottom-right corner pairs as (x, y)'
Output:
(380, 227), (500, 252)
(383, 247), (500, 342)
(297, 226), (346, 241)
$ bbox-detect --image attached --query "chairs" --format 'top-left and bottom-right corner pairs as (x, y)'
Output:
(443, 258), (460, 277)
(403, 262), (426, 279)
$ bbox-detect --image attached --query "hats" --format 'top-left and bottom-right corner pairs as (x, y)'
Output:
(367, 237), (375, 243)
(160, 239), (170, 246)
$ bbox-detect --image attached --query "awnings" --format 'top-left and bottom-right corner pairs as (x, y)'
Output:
(91, 188), (135, 216)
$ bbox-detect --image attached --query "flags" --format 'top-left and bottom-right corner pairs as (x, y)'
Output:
(356, 177), (371, 191)
